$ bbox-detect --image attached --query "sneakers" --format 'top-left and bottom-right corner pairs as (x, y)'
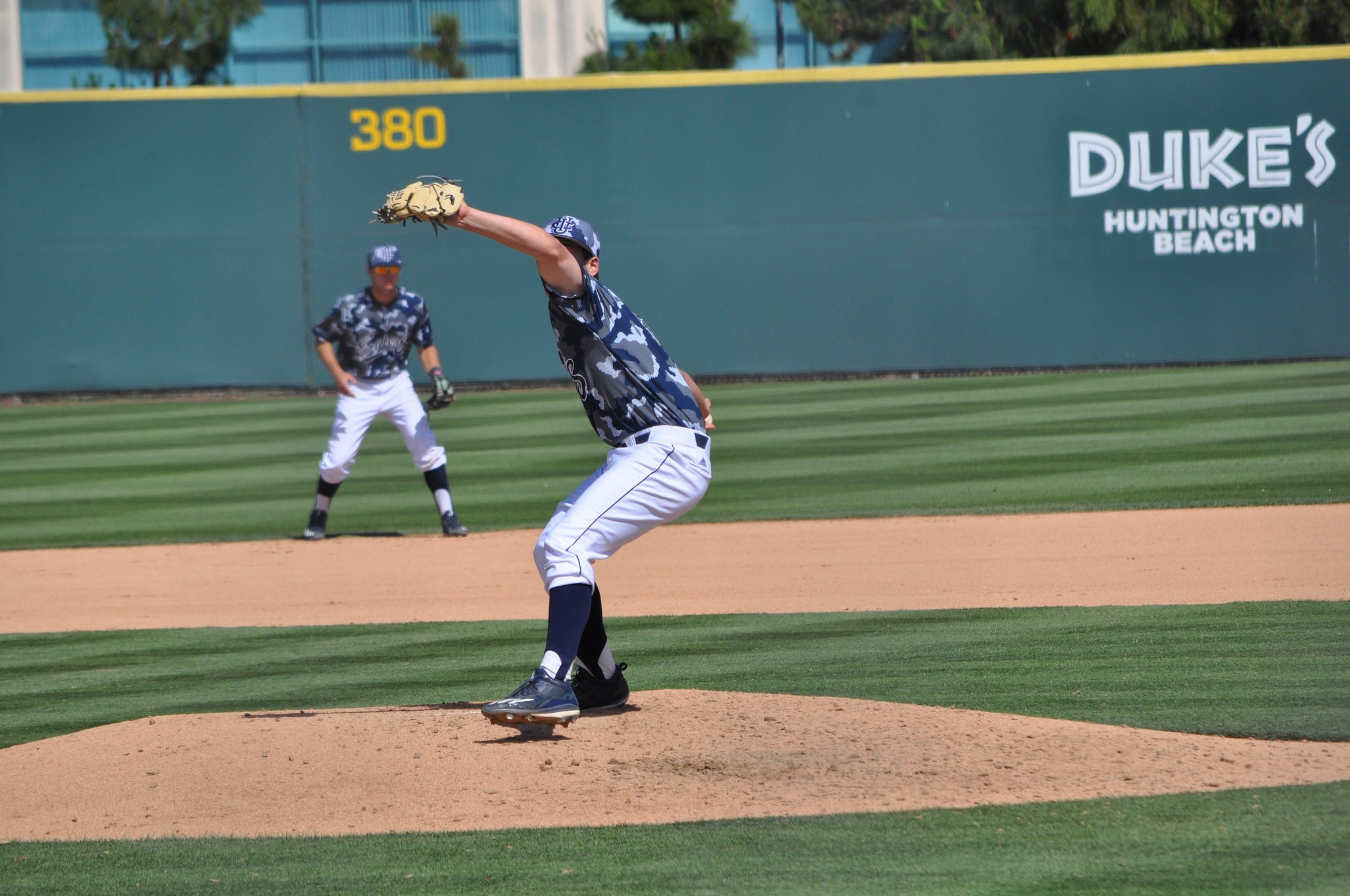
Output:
(481, 668), (580, 728)
(571, 662), (629, 712)
(304, 508), (328, 539)
(441, 510), (469, 537)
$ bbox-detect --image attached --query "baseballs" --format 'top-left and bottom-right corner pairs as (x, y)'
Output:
(705, 414), (713, 423)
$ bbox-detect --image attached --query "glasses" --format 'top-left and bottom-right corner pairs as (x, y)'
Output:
(372, 266), (400, 274)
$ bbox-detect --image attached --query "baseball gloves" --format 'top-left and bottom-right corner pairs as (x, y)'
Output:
(423, 373), (456, 426)
(377, 175), (466, 226)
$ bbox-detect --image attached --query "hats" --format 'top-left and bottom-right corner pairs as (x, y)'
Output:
(542, 215), (601, 258)
(367, 244), (403, 267)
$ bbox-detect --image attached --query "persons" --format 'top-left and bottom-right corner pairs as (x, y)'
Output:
(300, 246), (468, 539)
(380, 177), (718, 735)
